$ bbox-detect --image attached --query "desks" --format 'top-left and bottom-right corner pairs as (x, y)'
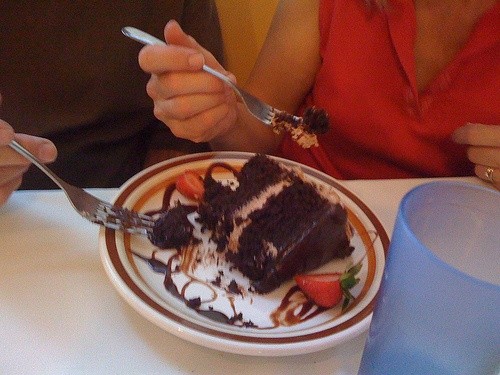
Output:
(0, 177), (500, 375)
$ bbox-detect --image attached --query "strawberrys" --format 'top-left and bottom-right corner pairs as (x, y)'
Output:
(292, 264), (362, 309)
(174, 171), (207, 201)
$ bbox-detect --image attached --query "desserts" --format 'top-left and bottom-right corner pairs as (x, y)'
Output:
(145, 153), (354, 294)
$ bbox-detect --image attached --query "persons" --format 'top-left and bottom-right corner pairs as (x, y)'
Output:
(138, 0), (500, 195)
(0, 0), (227, 209)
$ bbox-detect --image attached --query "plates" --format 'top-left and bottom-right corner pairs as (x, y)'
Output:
(99, 150), (392, 355)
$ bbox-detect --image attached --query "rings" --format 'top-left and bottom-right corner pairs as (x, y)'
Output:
(485, 168), (496, 184)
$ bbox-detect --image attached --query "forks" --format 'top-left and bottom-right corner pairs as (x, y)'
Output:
(8, 140), (158, 235)
(120, 25), (301, 128)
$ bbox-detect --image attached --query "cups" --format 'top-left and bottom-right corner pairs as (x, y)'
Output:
(357, 182), (500, 375)
